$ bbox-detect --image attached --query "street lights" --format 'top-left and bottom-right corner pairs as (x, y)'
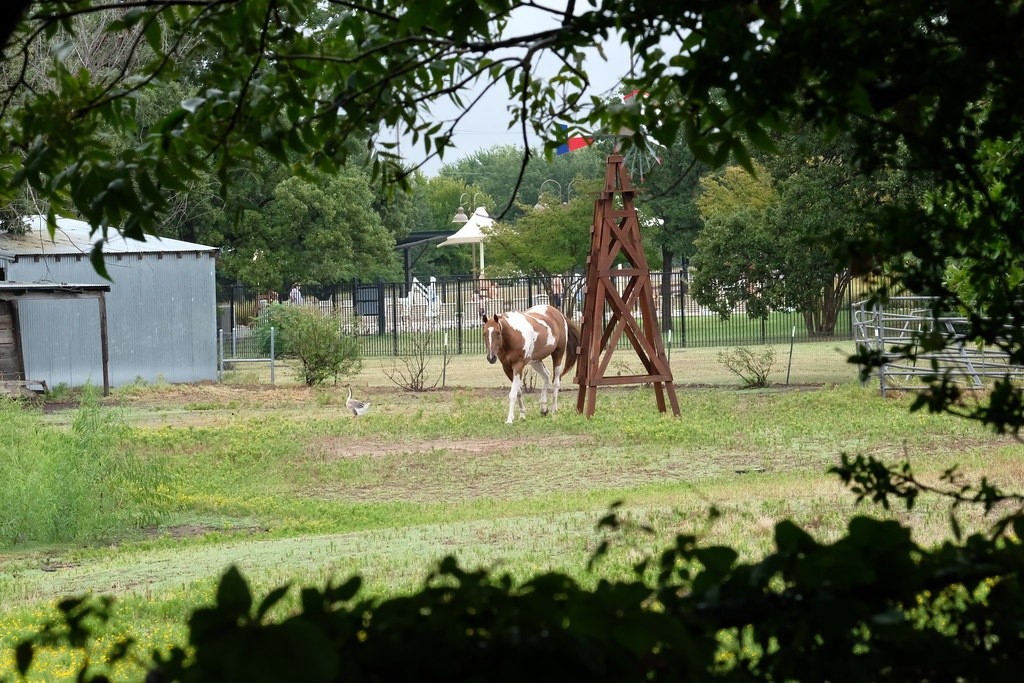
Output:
(451, 191), (491, 299)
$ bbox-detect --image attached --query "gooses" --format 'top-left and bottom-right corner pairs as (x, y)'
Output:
(340, 383), (371, 420)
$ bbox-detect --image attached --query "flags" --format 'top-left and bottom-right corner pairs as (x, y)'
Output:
(552, 120), (593, 157)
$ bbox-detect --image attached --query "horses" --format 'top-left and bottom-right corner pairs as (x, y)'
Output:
(482, 303), (581, 426)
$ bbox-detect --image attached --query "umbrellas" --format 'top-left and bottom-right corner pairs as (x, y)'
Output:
(436, 208), (519, 283)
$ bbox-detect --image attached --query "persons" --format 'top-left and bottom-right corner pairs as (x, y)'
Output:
(473, 282), (500, 304)
(288, 282), (304, 306)
(551, 271), (584, 312)
